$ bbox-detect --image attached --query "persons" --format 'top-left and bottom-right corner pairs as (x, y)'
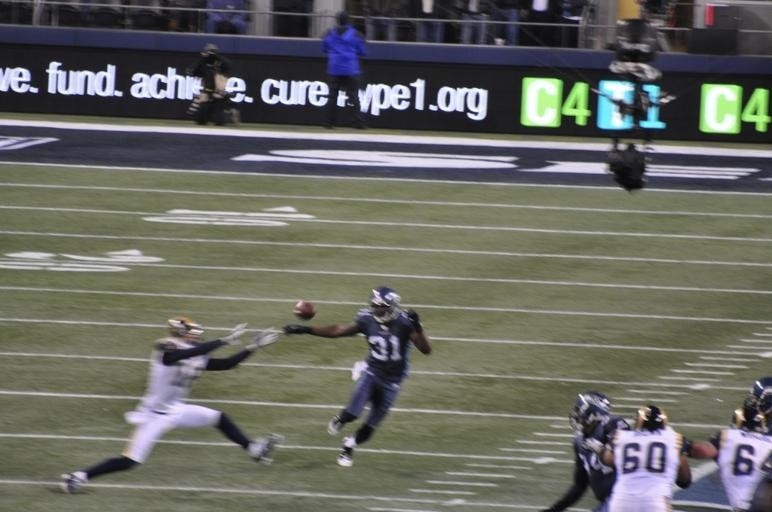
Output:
(190, 43), (238, 125)
(318, 11), (365, 129)
(31, 0), (250, 35)
(283, 286), (432, 468)
(543, 378), (772, 512)
(61, 317), (283, 496)
(344, 1), (595, 48)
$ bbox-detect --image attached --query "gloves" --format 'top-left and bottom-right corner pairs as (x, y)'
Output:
(283, 323), (314, 337)
(673, 431), (694, 458)
(245, 328), (279, 352)
(218, 322), (249, 347)
(400, 309), (425, 333)
(581, 435), (607, 458)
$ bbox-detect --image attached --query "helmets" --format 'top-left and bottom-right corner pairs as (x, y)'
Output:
(730, 405), (768, 434)
(368, 287), (402, 314)
(166, 315), (204, 346)
(632, 404), (667, 433)
(750, 377), (772, 415)
(572, 391), (612, 427)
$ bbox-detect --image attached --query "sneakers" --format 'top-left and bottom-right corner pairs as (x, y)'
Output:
(334, 436), (355, 468)
(325, 413), (344, 435)
(249, 429), (283, 470)
(60, 471), (87, 494)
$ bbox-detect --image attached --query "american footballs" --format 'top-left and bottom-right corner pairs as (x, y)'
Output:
(293, 300), (316, 320)
